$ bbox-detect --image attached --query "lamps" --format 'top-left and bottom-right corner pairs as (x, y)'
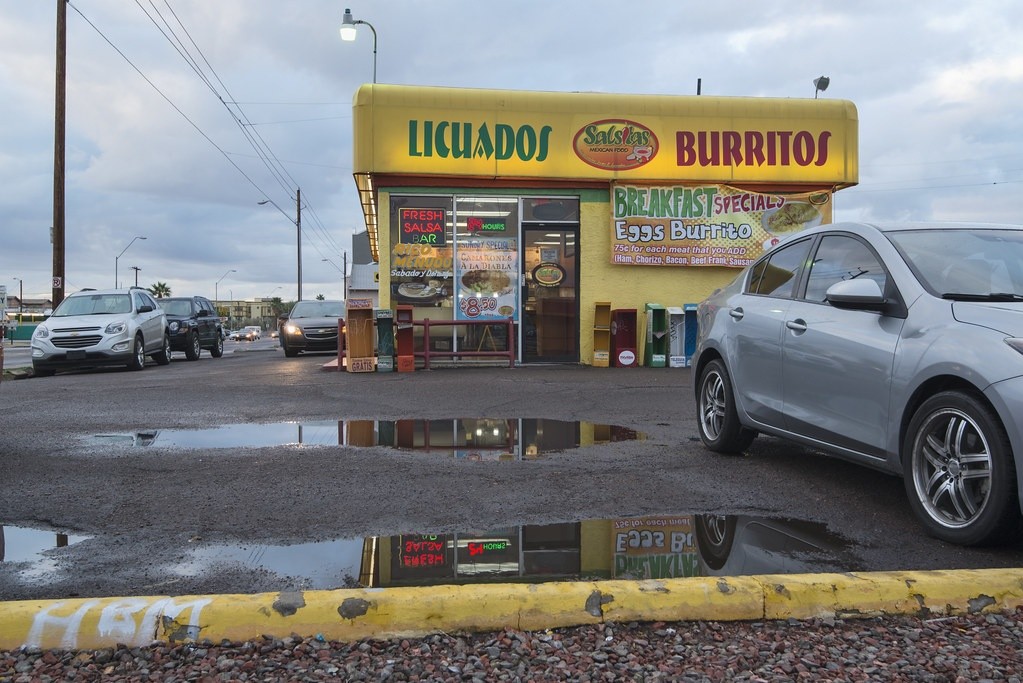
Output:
(340, 9), (356, 41)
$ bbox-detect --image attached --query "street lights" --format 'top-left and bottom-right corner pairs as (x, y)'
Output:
(266, 286), (282, 302)
(114, 235), (148, 291)
(12, 277), (23, 326)
(339, 7), (378, 86)
(215, 268), (238, 318)
(256, 199), (301, 301)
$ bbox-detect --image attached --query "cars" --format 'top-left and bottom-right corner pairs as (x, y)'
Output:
(693, 512), (860, 576)
(280, 299), (347, 358)
(30, 286), (173, 378)
(688, 222), (1023, 544)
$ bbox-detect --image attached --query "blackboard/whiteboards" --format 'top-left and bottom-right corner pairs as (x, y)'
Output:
(468, 540), (509, 559)
(467, 218), (507, 231)
(398, 535), (448, 571)
(399, 208), (446, 246)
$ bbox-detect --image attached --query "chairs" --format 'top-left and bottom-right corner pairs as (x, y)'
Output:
(947, 260), (990, 293)
(880, 252), (944, 299)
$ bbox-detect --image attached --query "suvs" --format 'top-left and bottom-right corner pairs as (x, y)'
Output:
(155, 295), (223, 362)
